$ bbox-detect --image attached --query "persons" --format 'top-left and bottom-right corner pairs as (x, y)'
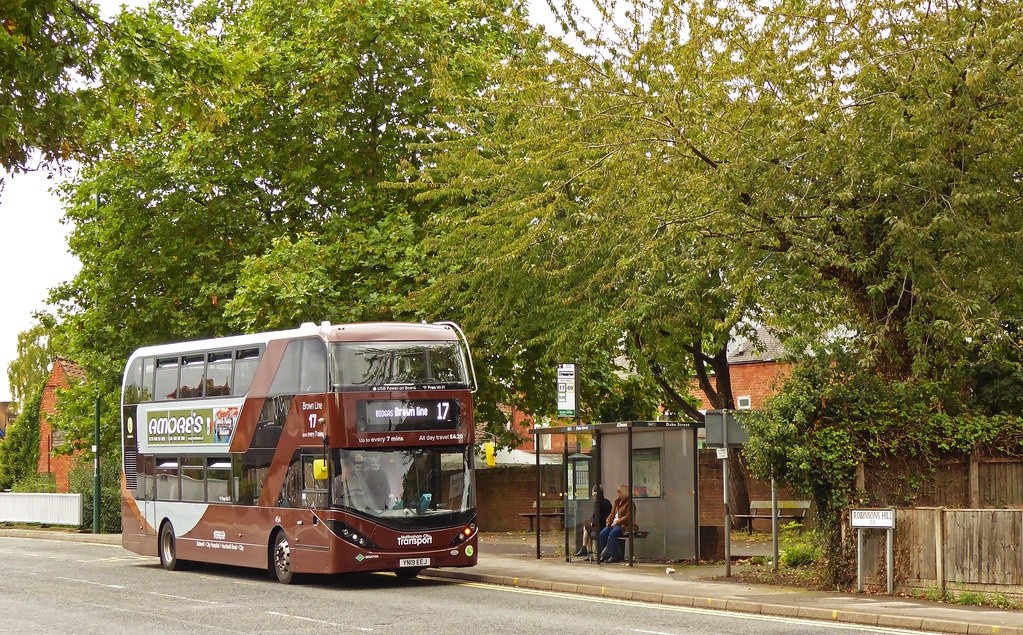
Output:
(573, 486), (612, 561)
(600, 485), (636, 564)
(332, 457), (377, 510)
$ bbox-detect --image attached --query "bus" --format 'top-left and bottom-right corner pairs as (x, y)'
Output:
(118, 315), (498, 587)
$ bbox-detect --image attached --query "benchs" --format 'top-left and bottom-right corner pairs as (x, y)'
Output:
(591, 530), (650, 539)
(518, 501), (577, 532)
(735, 500), (811, 535)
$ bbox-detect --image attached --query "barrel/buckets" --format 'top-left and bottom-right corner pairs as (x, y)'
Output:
(700, 525), (725, 563)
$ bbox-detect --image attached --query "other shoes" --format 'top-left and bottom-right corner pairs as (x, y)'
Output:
(584, 554), (593, 561)
(599, 557), (606, 562)
(606, 556), (620, 563)
(574, 547), (587, 556)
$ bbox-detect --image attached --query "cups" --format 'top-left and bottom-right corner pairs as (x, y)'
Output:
(666, 568), (675, 574)
